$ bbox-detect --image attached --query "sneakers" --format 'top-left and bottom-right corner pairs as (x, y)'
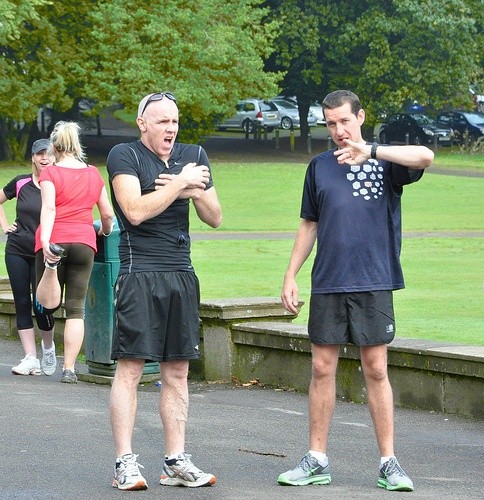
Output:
(41, 339), (57, 376)
(12, 356), (41, 375)
(112, 454), (148, 490)
(277, 453), (332, 486)
(377, 457), (414, 491)
(160, 452), (216, 487)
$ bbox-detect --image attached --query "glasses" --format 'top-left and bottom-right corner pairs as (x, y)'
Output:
(141, 93), (176, 117)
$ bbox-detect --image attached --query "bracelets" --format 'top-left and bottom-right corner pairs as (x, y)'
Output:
(101, 230), (112, 237)
(371, 142), (378, 159)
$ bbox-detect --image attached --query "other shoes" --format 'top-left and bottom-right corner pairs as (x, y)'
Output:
(47, 242), (65, 266)
(61, 369), (78, 384)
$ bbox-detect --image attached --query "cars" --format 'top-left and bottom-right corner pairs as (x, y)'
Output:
(379, 111), (484, 147)
(216, 96), (327, 133)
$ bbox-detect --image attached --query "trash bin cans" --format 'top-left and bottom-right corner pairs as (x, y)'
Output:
(85, 218), (160, 376)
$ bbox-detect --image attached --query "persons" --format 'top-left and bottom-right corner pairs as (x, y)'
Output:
(278, 89), (436, 492)
(106, 93), (222, 489)
(0, 138), (57, 376)
(34, 116), (115, 383)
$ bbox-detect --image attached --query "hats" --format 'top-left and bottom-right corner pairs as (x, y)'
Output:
(32, 138), (50, 154)
